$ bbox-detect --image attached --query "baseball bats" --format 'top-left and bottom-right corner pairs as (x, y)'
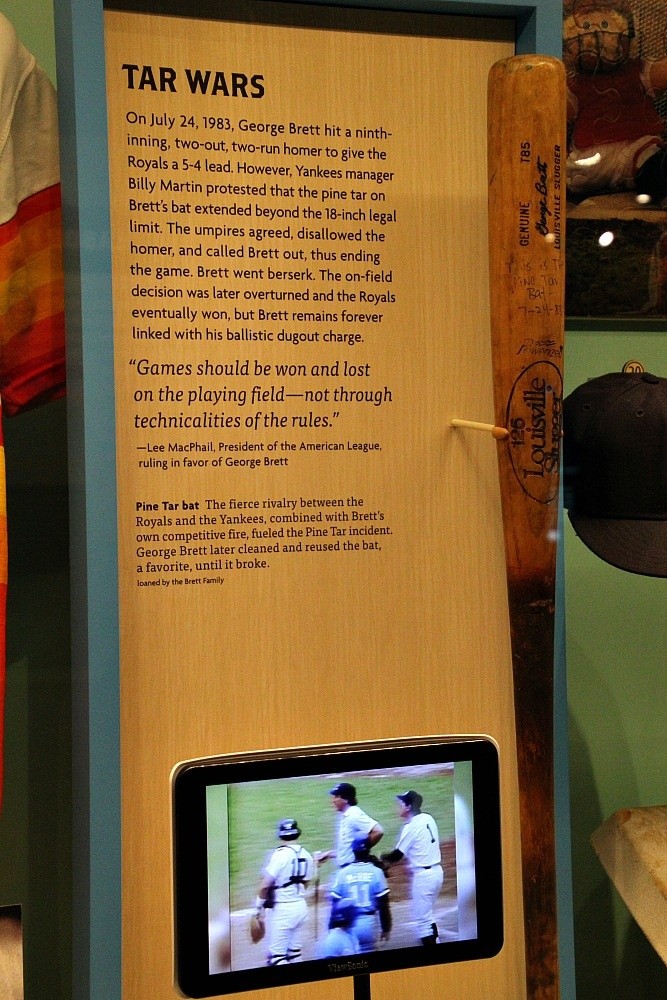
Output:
(487, 54), (567, 1000)
(314, 850), (321, 939)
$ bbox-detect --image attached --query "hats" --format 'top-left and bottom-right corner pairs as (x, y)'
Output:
(396, 790), (422, 808)
(330, 783), (356, 798)
(350, 833), (370, 850)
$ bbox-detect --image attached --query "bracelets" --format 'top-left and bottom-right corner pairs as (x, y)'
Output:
(256, 895), (268, 908)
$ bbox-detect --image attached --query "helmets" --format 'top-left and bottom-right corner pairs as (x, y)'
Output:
(278, 818), (301, 836)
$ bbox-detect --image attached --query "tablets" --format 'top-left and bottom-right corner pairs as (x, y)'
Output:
(172, 734), (505, 999)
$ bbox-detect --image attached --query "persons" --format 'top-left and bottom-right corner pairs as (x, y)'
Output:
(313, 782), (391, 959)
(255, 819), (316, 966)
(380, 790), (443, 946)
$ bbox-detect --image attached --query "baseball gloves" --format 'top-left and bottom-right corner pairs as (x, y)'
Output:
(251, 916), (265, 944)
